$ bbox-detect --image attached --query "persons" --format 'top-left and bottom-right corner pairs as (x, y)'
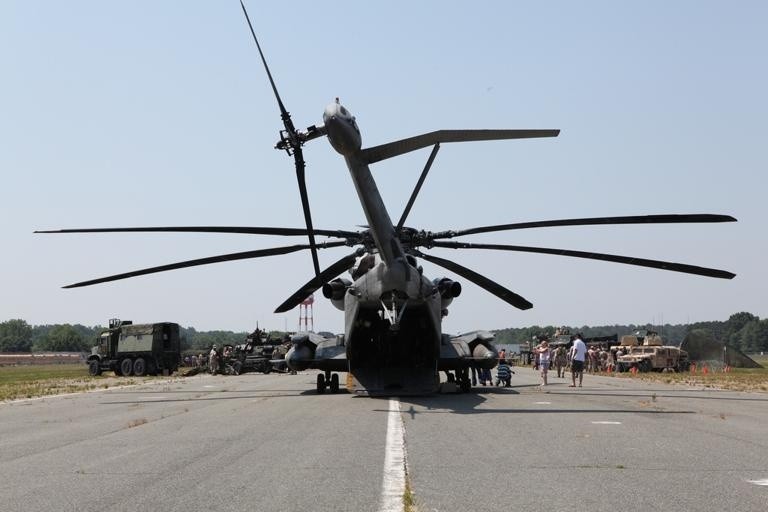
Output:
(471, 366), (483, 386)
(225, 346), (233, 357)
(185, 351), (204, 367)
(271, 346), (282, 372)
(482, 367), (494, 386)
(568, 333), (587, 387)
(534, 325), (611, 378)
(532, 340), (550, 387)
(497, 359), (515, 387)
(210, 343), (220, 375)
(498, 347), (506, 360)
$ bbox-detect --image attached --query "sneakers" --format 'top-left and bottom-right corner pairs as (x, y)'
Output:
(568, 384), (582, 387)
(483, 382), (510, 386)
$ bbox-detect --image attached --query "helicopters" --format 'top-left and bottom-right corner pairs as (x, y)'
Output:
(33, 0), (738, 397)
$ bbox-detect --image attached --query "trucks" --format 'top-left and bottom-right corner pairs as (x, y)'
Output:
(85, 318), (181, 377)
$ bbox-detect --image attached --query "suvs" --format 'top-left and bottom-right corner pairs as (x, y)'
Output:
(616, 345), (689, 373)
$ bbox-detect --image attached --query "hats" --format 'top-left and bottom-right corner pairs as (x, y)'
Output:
(213, 345), (217, 349)
